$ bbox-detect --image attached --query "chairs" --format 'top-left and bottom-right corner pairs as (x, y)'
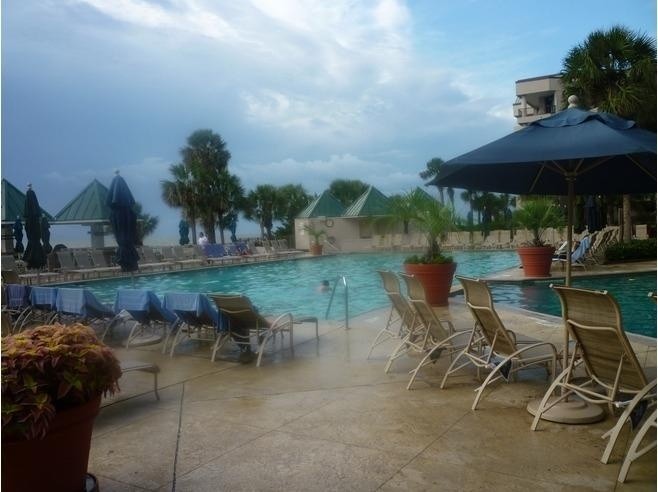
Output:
(532, 284), (654, 432)
(44, 288), (116, 327)
(386, 273), (485, 390)
(1, 237), (301, 282)
(1, 286), (36, 332)
(398, 273), (428, 300)
(162, 293), (231, 358)
(553, 227), (622, 270)
(117, 360), (164, 403)
(365, 270), (455, 359)
(211, 295), (318, 367)
(101, 287), (178, 350)
(18, 286), (58, 332)
(441, 276), (565, 413)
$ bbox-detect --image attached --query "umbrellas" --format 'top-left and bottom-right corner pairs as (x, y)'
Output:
(106, 166), (141, 288)
(13, 216), (24, 265)
(23, 182), (46, 286)
(425, 95), (657, 289)
(41, 214), (52, 254)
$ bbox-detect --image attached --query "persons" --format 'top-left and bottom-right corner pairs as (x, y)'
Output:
(316, 279), (332, 292)
(197, 232), (208, 250)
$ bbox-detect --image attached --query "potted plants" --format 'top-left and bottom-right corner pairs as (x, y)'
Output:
(401, 188), (458, 307)
(602, 380), (656, 482)
(2, 324), (123, 489)
(509, 194), (566, 277)
(302, 220), (330, 258)
